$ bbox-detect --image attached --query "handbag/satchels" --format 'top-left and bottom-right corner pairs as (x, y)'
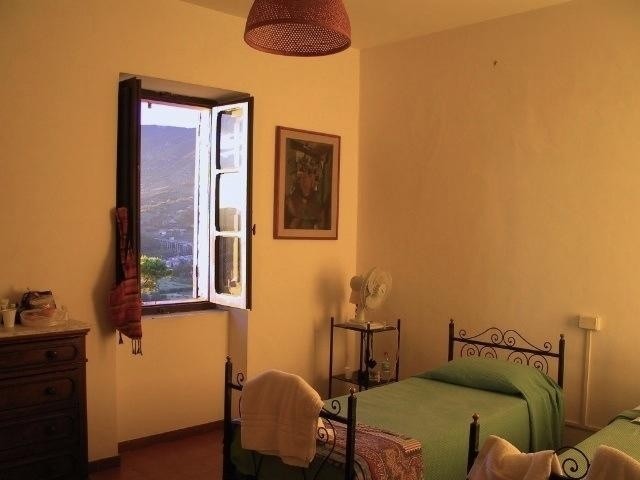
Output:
(104, 207), (143, 340)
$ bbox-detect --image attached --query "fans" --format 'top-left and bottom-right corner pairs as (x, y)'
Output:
(346, 265), (391, 331)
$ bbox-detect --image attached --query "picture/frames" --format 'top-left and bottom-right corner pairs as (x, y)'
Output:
(270, 125), (341, 241)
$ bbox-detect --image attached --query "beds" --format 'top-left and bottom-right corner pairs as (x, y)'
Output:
(223, 320), (568, 479)
(467, 405), (640, 480)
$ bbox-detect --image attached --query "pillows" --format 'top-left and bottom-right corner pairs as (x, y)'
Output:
(418, 356), (540, 398)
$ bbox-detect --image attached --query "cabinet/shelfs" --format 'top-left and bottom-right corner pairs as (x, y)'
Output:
(0, 319), (93, 480)
(328, 316), (401, 399)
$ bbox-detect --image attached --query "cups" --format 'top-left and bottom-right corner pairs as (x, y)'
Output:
(344, 366), (353, 380)
(3, 308), (16, 329)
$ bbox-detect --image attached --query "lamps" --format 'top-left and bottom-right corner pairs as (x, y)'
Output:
(244, 0), (352, 56)
